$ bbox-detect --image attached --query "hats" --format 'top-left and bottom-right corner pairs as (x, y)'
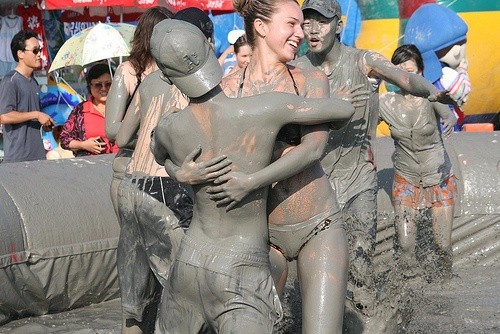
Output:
(149, 18), (222, 98)
(301, 0), (342, 19)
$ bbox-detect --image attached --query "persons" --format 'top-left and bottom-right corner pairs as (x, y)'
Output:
(60, 64), (120, 157)
(163, 1), (355, 334)
(0, 29), (55, 161)
(381, 44), (457, 267)
(105, 5), (174, 334)
(403, 0), (471, 132)
(289, 0), (461, 274)
(150, 18), (356, 334)
(117, 7), (214, 334)
(224, 35), (251, 76)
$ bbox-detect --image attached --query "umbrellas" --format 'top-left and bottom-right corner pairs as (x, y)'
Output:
(47, 24), (138, 73)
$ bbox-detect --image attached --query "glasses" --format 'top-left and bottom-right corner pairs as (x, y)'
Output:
(89, 82), (112, 88)
(22, 48), (43, 53)
